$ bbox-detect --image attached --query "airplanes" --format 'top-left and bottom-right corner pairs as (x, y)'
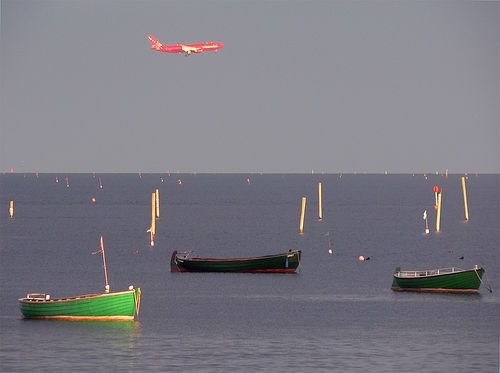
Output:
(145, 32), (225, 56)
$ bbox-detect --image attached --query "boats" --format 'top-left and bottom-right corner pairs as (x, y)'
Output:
(17, 284), (141, 322)
(392, 262), (493, 295)
(169, 248), (302, 274)
